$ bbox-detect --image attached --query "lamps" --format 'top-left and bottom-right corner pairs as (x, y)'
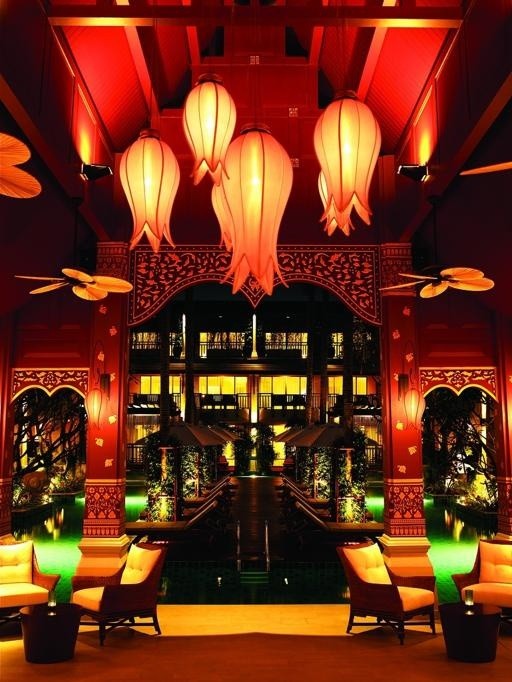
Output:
(82, 161), (113, 182)
(84, 339), (113, 429)
(397, 338), (427, 433)
(397, 164), (427, 182)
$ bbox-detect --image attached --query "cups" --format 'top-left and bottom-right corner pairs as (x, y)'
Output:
(464, 587), (475, 607)
(48, 588), (57, 609)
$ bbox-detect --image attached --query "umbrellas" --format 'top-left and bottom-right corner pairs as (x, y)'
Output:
(133, 415), (381, 523)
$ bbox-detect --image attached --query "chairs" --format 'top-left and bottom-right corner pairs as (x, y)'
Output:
(335, 539), (436, 646)
(450, 538), (512, 636)
(69, 542), (169, 647)
(124, 476), (232, 537)
(279, 472), (384, 543)
(0, 540), (62, 636)
